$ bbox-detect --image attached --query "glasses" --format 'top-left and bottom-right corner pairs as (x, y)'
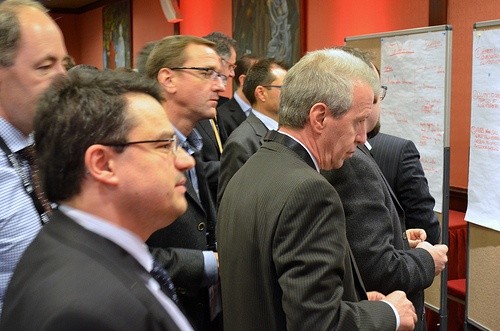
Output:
(380, 85), (388, 100)
(220, 54), (238, 72)
(170, 66), (227, 86)
(121, 137), (181, 152)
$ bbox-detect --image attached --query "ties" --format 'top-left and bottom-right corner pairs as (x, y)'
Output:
(25, 146), (53, 217)
(149, 262), (180, 307)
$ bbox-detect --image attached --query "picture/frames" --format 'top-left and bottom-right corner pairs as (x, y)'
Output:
(232, 0), (307, 71)
(101, 1), (133, 73)
